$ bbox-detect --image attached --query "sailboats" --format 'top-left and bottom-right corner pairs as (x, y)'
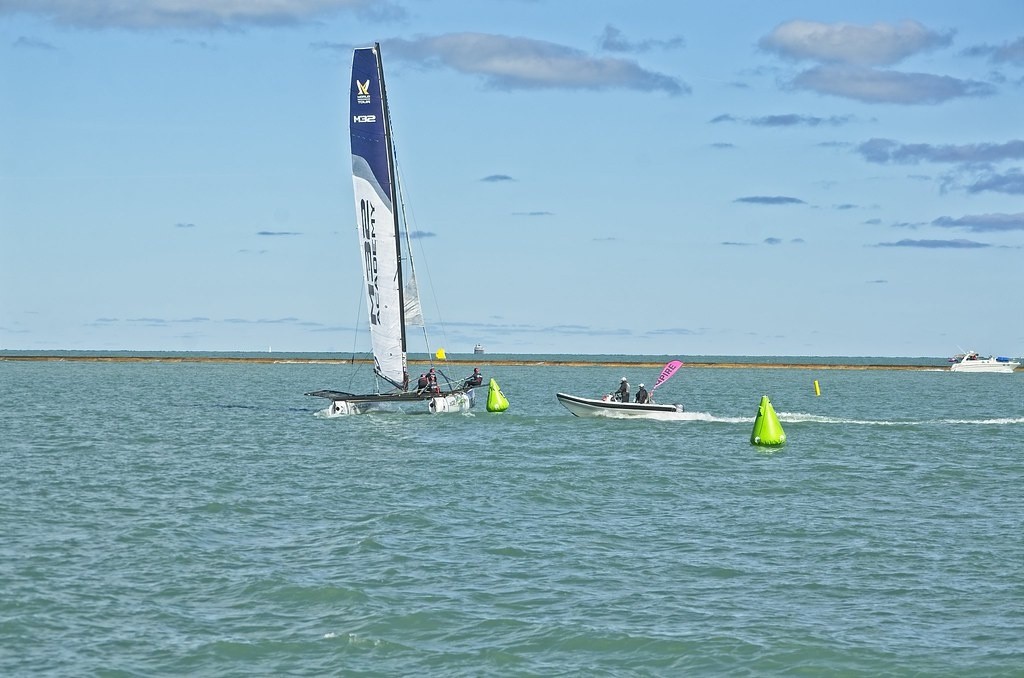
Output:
(301, 41), (490, 418)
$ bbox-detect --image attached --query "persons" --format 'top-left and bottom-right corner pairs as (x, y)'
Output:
(463, 368), (483, 388)
(635, 383), (649, 404)
(615, 377), (630, 401)
(417, 368), (440, 394)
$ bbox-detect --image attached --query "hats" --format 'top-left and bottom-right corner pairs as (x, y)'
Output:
(621, 377), (626, 381)
(639, 383), (644, 387)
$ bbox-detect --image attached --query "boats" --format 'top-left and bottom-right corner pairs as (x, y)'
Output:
(474, 342), (485, 354)
(951, 346), (1022, 373)
(556, 392), (685, 421)
